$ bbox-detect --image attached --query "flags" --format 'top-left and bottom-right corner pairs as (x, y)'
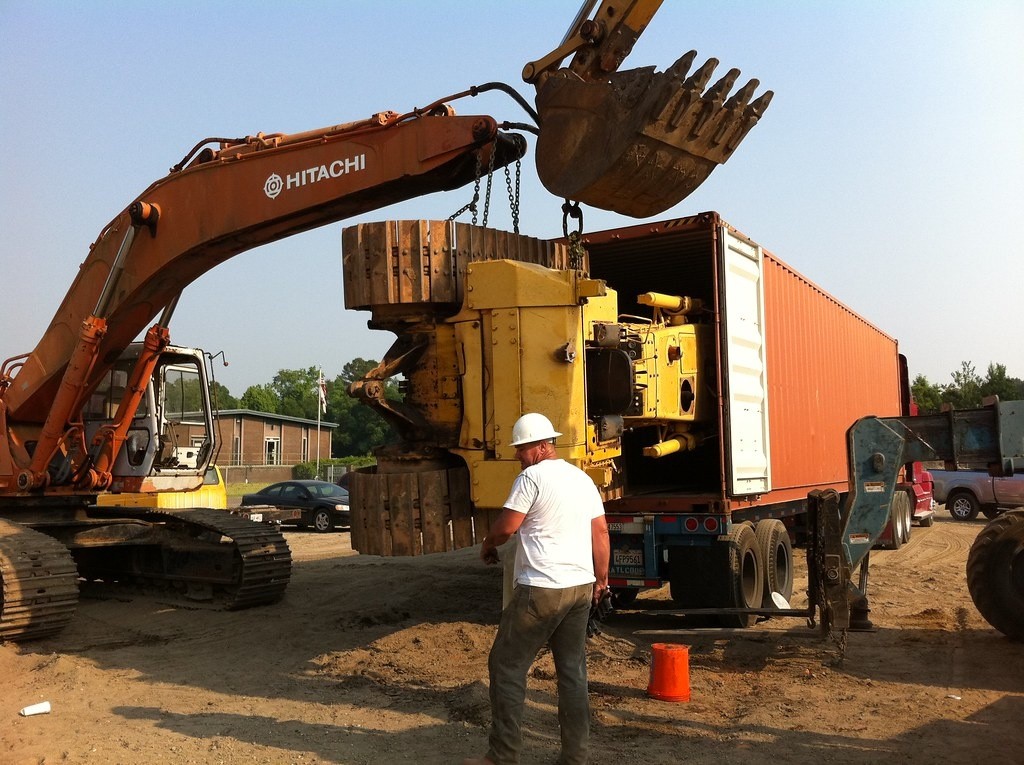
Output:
(320, 377), (327, 413)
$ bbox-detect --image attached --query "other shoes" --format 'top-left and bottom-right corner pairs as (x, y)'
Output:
(464, 756), (495, 765)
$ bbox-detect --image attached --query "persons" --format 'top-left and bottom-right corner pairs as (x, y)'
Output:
(463, 414), (611, 765)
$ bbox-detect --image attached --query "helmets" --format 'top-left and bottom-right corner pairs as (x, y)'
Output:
(508, 412), (563, 446)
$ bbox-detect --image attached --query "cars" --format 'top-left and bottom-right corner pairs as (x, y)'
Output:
(240, 477), (351, 533)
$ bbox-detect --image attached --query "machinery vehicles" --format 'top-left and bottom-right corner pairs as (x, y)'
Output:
(339, 218), (716, 558)
(809, 392), (1024, 641)
(1, 1), (775, 643)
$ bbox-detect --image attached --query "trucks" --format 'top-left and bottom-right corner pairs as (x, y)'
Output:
(542, 209), (939, 625)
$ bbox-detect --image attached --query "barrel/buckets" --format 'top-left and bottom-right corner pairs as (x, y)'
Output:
(644, 643), (691, 702)
